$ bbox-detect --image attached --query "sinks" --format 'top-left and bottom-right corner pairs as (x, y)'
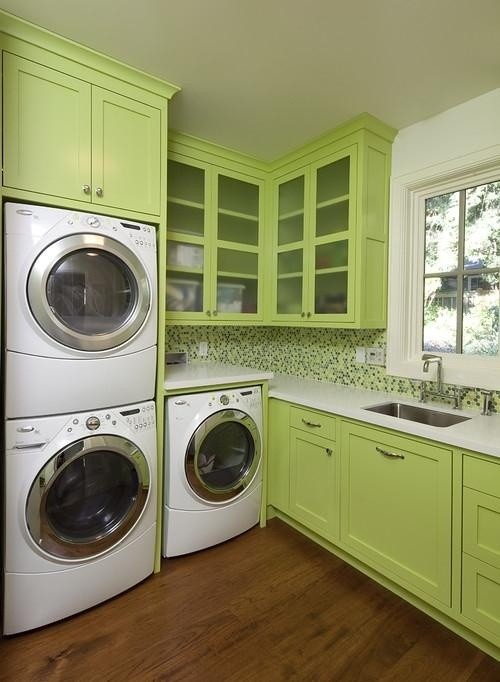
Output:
(360, 399), (472, 428)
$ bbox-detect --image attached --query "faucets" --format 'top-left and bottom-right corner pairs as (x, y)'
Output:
(422, 356), (447, 393)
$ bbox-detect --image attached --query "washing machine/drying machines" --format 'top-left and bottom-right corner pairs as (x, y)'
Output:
(0, 201), (159, 422)
(161, 384), (264, 558)
(0, 399), (160, 636)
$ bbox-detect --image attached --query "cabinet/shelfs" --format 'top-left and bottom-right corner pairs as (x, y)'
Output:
(339, 416), (455, 640)
(165, 127), (263, 326)
(263, 114), (398, 329)
(1, 9), (181, 223)
(456, 447), (500, 665)
(268, 397), (339, 555)
